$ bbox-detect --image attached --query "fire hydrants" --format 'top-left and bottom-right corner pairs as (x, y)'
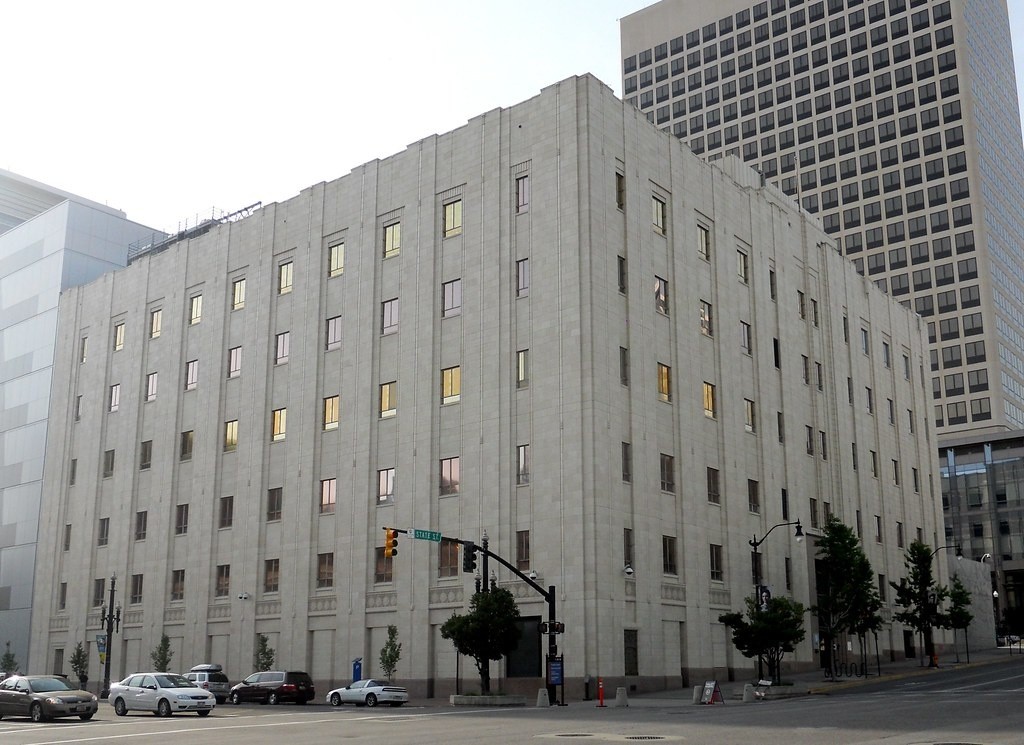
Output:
(933, 654), (939, 669)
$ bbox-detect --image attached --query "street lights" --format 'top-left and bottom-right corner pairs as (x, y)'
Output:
(920, 544), (963, 666)
(101, 571), (122, 700)
(749, 519), (805, 685)
(993, 590), (1000, 635)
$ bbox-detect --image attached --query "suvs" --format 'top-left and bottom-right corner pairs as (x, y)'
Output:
(229, 671), (315, 705)
(182, 663), (231, 705)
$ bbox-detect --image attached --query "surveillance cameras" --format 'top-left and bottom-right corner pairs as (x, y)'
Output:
(530, 573), (536, 580)
(243, 594), (248, 599)
(987, 554), (991, 559)
(626, 568), (633, 575)
(238, 594), (242, 599)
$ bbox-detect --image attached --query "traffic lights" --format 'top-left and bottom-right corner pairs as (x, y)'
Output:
(463, 541), (477, 574)
(385, 528), (398, 558)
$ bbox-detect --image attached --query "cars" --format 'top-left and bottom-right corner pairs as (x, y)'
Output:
(0, 675), (98, 722)
(325, 679), (408, 707)
(108, 672), (216, 717)
